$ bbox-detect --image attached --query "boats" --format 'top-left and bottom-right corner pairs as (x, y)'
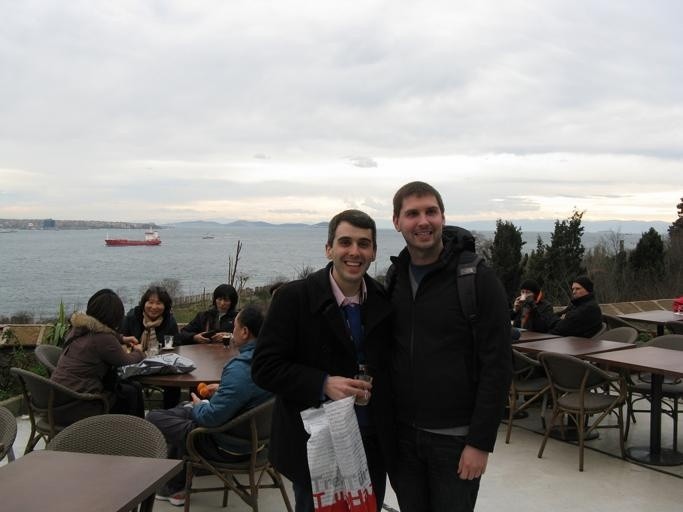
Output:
(103, 225), (163, 248)
(200, 235), (214, 239)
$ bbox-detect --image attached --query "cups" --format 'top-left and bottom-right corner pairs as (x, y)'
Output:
(220, 336), (231, 351)
(353, 374), (372, 406)
(164, 335), (173, 347)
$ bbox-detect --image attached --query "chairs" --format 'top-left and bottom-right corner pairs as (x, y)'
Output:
(505, 312), (683, 473)
(8, 367), (109, 455)
(33, 344), (64, 378)
(181, 394), (294, 512)
(45, 413), (168, 511)
(0, 404), (19, 463)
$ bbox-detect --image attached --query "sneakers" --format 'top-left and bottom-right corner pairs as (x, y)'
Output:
(153, 489), (187, 506)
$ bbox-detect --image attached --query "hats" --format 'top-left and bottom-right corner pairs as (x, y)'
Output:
(573, 277), (594, 292)
(521, 279), (541, 294)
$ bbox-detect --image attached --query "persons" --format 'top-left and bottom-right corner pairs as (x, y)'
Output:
(508, 283), (556, 405)
(251, 210), (398, 511)
(381, 182), (512, 512)
(551, 277), (602, 340)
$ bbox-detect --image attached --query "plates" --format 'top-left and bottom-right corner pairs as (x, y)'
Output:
(160, 347), (175, 351)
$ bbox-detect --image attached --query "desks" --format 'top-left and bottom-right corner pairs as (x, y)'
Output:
(615, 309), (683, 336)
(127, 342), (241, 412)
(0, 448), (186, 511)
